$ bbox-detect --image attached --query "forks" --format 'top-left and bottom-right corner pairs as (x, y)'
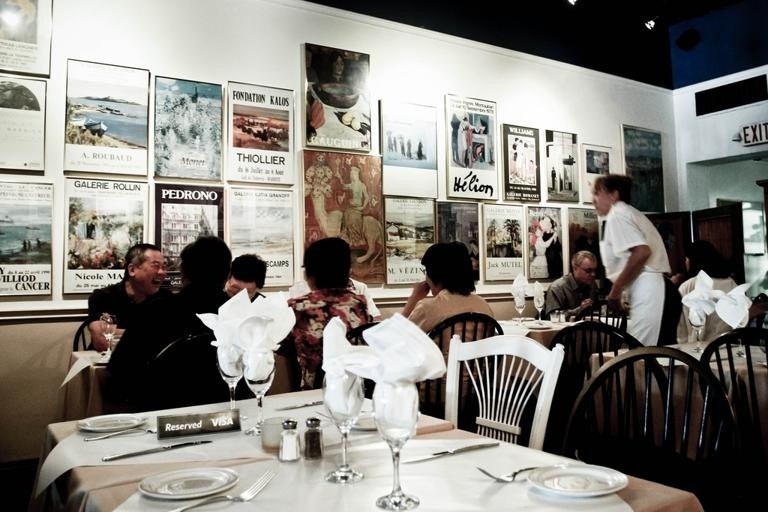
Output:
(477, 463), (544, 485)
(168, 468), (276, 512)
(82, 420), (158, 443)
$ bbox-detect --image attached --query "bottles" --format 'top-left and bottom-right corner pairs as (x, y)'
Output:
(278, 417), (324, 464)
(552, 308), (565, 323)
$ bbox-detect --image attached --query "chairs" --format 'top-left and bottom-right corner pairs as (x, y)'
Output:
(549, 320), (675, 487)
(313, 321), (380, 399)
(144, 333), (252, 410)
(562, 346), (745, 510)
(698, 328), (766, 511)
(413, 311), (504, 436)
(72, 317), (96, 350)
(445, 331), (565, 451)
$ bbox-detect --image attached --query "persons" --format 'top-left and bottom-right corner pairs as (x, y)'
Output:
(403, 241), (495, 408)
(82, 236), (383, 415)
(549, 166), (557, 190)
(666, 239), (737, 346)
(386, 134), (424, 159)
(534, 217), (563, 279)
(303, 150), (384, 249)
(511, 135), (536, 183)
(591, 174), (685, 363)
(544, 249), (602, 320)
(529, 221), (558, 280)
(319, 50), (358, 88)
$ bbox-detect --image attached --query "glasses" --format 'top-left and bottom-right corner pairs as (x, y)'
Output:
(582, 267), (597, 272)
(228, 279), (257, 296)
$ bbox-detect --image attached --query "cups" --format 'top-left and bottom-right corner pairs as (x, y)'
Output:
(261, 414), (286, 455)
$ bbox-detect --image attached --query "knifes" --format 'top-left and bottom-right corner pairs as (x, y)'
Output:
(276, 399), (322, 414)
(102, 437), (212, 462)
(707, 356), (729, 363)
(400, 440), (499, 466)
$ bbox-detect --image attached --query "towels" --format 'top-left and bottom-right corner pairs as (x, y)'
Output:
(681, 268), (752, 330)
(508, 272), (545, 306)
(194, 289), (447, 442)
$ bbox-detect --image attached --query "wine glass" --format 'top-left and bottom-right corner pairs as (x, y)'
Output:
(370, 380), (422, 512)
(685, 303), (707, 354)
(511, 287), (528, 326)
(321, 368), (367, 486)
(215, 342), (277, 439)
(98, 315), (118, 362)
(533, 288), (546, 322)
(731, 306), (750, 360)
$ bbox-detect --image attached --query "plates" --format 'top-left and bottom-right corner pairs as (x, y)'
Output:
(136, 466), (241, 501)
(76, 413), (146, 434)
(352, 411), (377, 431)
(527, 323), (552, 330)
(527, 464), (628, 497)
